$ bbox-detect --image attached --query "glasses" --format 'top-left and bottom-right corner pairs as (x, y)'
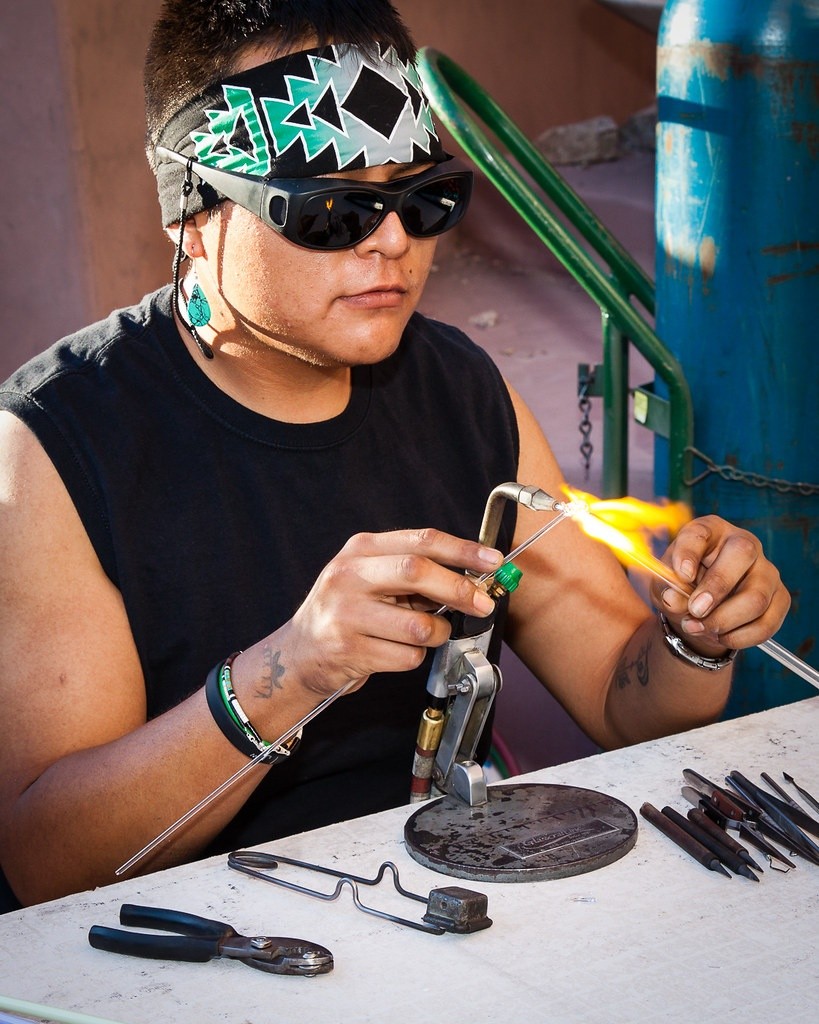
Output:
(154, 147), (472, 252)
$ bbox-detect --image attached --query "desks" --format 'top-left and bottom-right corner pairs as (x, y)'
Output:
(1, 690), (818, 1024)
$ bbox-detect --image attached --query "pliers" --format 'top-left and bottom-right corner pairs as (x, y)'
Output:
(90, 903), (336, 979)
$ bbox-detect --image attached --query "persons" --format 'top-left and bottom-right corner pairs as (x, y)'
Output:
(0, 0), (793, 915)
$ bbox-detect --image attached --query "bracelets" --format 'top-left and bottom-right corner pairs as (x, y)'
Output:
(217, 650), (303, 758)
(657, 609), (741, 673)
(204, 660), (303, 765)
